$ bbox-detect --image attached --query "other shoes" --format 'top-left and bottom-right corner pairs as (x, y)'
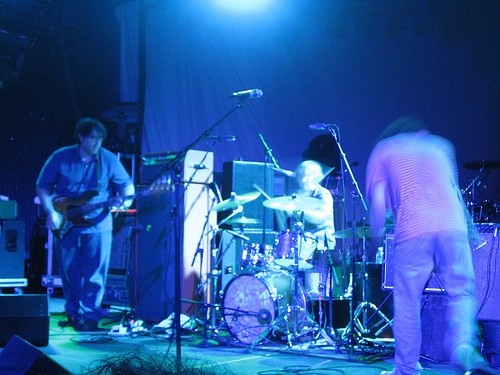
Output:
(451, 345), (490, 375)
(76, 319), (97, 332)
(58, 318), (75, 327)
(379, 368), (412, 375)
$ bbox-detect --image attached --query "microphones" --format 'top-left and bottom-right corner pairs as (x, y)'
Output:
(233, 88), (264, 99)
(207, 135), (236, 140)
(309, 124), (326, 130)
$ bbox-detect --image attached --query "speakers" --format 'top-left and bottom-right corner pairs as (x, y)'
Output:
(222, 161), (274, 228)
(0, 292), (49, 348)
(0, 334), (75, 375)
(135, 182), (217, 321)
(466, 226), (500, 321)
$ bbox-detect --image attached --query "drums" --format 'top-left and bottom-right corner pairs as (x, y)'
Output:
(222, 265), (308, 346)
(239, 243), (277, 276)
(302, 245), (354, 303)
(272, 228), (318, 272)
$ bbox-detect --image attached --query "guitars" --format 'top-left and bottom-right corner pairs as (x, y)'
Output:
(50, 189), (151, 241)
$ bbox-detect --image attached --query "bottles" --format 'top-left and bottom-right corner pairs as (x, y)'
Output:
(376, 247), (384, 264)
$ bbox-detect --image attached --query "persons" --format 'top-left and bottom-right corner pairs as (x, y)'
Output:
(35, 118), (137, 333)
(286, 161), (336, 299)
(361, 115), (499, 375)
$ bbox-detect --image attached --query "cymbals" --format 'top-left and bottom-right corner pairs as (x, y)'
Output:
(262, 196), (325, 210)
(211, 189), (264, 212)
(221, 216), (263, 228)
(329, 224), (390, 239)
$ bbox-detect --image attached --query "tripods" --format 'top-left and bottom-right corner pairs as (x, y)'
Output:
(148, 98), (393, 355)
